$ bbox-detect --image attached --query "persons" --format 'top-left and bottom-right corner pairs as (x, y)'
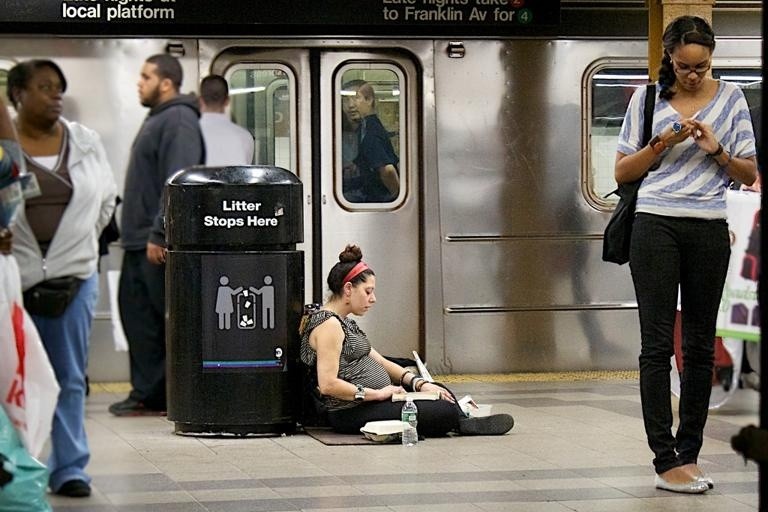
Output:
(613, 13), (757, 494)
(0, 89), (24, 304)
(295, 244), (514, 436)
(106, 53), (205, 414)
(341, 78), (399, 202)
(197, 73), (254, 169)
(6, 58), (118, 498)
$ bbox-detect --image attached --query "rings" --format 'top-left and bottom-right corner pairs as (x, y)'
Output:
(671, 120), (680, 133)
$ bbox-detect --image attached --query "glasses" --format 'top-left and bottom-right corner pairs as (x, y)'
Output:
(672, 57), (714, 77)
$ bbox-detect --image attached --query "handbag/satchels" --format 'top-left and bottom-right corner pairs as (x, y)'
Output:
(21, 273), (84, 321)
(601, 174), (640, 267)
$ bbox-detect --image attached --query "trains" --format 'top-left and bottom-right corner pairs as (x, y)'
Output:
(0, 0), (767, 382)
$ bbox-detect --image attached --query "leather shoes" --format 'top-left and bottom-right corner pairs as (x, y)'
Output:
(654, 470), (716, 495)
(54, 480), (93, 499)
(107, 394), (169, 417)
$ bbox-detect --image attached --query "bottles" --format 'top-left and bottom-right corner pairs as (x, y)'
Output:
(401, 398), (419, 448)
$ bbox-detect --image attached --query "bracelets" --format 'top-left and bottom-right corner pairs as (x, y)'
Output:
(354, 383), (365, 403)
(706, 141), (723, 157)
(412, 378), (423, 392)
(409, 376), (420, 389)
(716, 150), (732, 168)
(400, 370), (409, 386)
(417, 380), (429, 392)
(648, 134), (671, 157)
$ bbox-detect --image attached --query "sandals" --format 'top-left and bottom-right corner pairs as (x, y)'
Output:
(455, 411), (515, 436)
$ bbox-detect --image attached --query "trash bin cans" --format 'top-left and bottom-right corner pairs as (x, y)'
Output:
(164, 165), (304, 439)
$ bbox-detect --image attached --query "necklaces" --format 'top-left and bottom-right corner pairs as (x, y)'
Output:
(672, 81), (709, 112)
